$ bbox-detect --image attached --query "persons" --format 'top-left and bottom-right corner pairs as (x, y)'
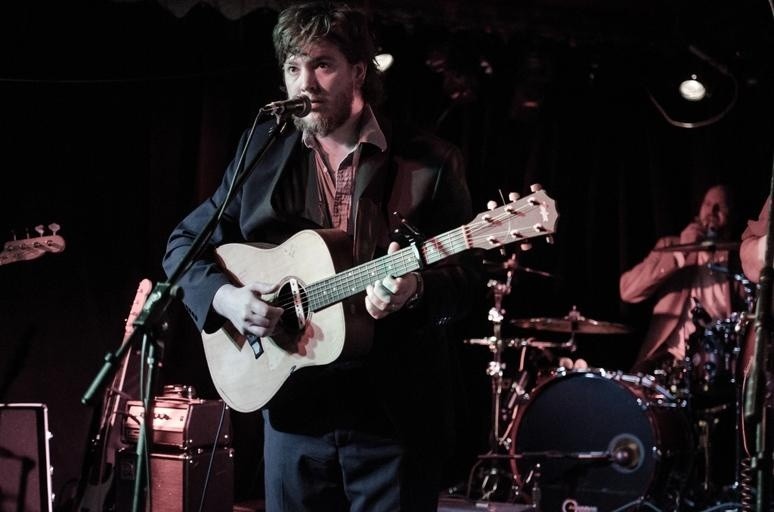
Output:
(619, 183), (774, 373)
(163, 0), (534, 512)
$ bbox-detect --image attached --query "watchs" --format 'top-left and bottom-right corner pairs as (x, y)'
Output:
(407, 272), (423, 304)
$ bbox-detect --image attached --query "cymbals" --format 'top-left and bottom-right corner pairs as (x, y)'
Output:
(480, 261), (552, 279)
(509, 317), (624, 334)
(655, 236), (740, 253)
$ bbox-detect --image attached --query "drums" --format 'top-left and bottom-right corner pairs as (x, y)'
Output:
(657, 311), (751, 428)
(510, 372), (698, 509)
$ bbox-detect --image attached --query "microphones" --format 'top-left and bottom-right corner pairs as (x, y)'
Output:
(259, 92), (313, 118)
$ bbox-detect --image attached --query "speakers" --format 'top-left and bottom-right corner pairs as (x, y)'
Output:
(113, 447), (234, 512)
(0, 401), (58, 512)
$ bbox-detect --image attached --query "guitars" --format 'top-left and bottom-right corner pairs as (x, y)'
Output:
(201, 183), (559, 412)
(60, 279), (153, 511)
(0, 223), (64, 265)
